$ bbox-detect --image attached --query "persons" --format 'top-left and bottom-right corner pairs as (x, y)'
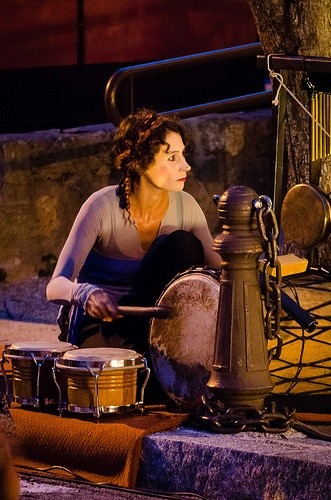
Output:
(46, 107), (224, 405)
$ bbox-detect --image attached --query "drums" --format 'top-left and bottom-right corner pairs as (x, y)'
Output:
(52, 347), (151, 420)
(0, 340), (77, 408)
(148, 265), (222, 412)
